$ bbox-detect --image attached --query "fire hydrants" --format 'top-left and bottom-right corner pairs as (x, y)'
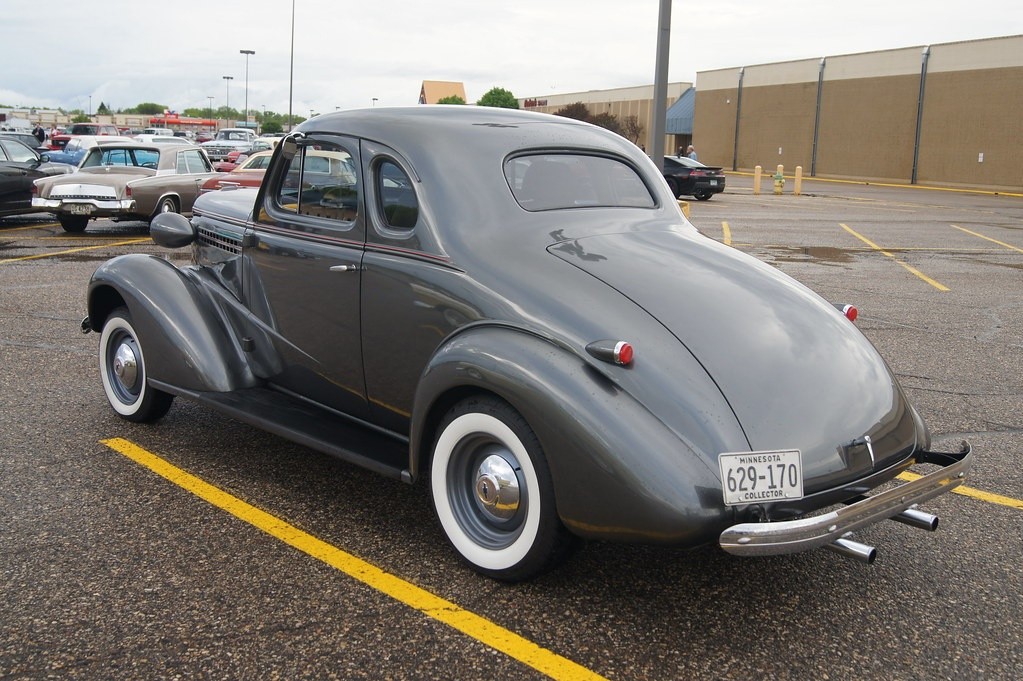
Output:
(773, 171), (785, 194)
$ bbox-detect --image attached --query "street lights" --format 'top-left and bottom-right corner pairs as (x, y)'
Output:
(310, 110), (313, 116)
(222, 75), (233, 128)
(372, 97), (379, 106)
(239, 49), (255, 129)
(261, 104), (266, 114)
(207, 96), (215, 132)
(335, 106), (340, 112)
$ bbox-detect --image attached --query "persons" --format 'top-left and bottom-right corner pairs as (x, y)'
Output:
(50, 124), (57, 138)
(641, 144), (645, 153)
(32, 122), (45, 146)
(686, 145), (697, 161)
(678, 146), (684, 156)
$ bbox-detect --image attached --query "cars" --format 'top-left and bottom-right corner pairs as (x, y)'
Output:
(0, 136), (77, 221)
(30, 140), (233, 232)
(616, 155), (726, 201)
(0, 117), (317, 166)
(73, 102), (975, 584)
(193, 148), (404, 199)
